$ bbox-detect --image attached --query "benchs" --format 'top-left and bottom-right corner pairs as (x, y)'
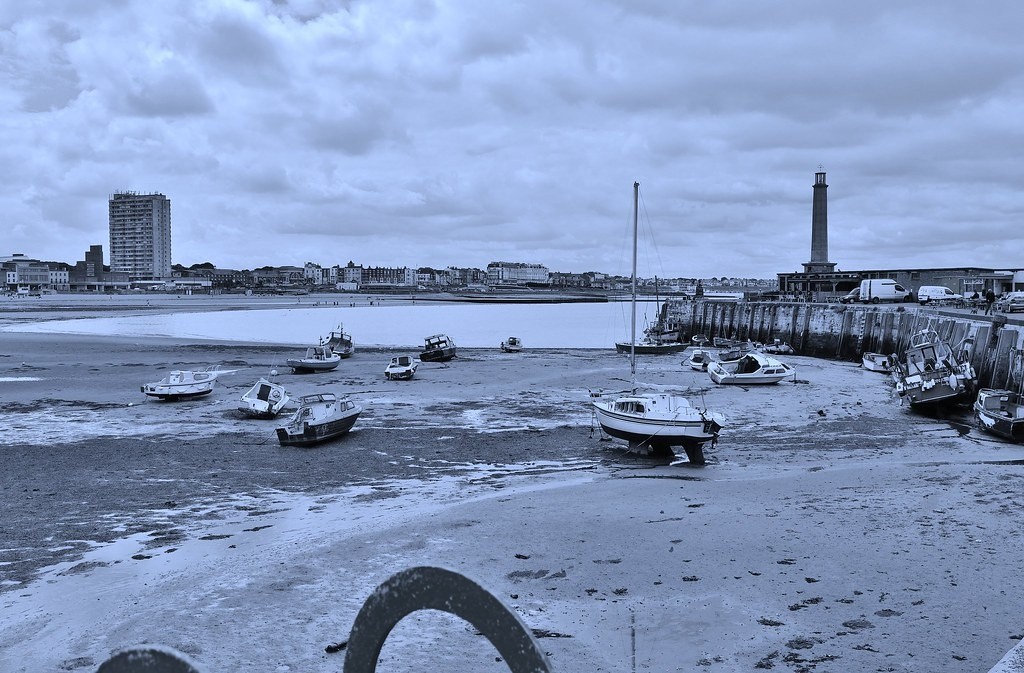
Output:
(825, 299), (839, 301)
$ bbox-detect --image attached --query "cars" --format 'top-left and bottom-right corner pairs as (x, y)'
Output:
(839, 287), (861, 304)
(1000, 292), (1024, 313)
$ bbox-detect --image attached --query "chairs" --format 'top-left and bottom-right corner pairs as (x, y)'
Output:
(779, 292), (818, 303)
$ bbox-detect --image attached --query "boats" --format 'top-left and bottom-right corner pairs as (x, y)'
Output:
(286, 336), (341, 374)
(862, 351), (896, 372)
(322, 320), (356, 359)
(891, 328), (971, 410)
(275, 391), (363, 447)
(419, 333), (458, 362)
(501, 336), (523, 352)
(707, 349), (796, 385)
(690, 340), (757, 372)
(384, 355), (418, 381)
(690, 331), (796, 355)
(973, 387), (1024, 442)
(237, 351), (289, 421)
(140, 364), (222, 403)
(616, 274), (691, 355)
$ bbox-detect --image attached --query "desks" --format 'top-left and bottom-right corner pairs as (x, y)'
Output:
(826, 296), (840, 304)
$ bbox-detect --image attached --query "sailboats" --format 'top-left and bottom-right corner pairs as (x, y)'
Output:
(590, 179), (727, 446)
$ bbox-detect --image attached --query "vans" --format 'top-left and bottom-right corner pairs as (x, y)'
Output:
(859, 279), (911, 304)
(918, 285), (963, 306)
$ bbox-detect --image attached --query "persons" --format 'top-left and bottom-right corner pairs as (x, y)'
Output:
(758, 289), (763, 302)
(970, 289), (979, 313)
(781, 289), (812, 302)
(995, 293), (1011, 299)
(985, 288), (995, 315)
